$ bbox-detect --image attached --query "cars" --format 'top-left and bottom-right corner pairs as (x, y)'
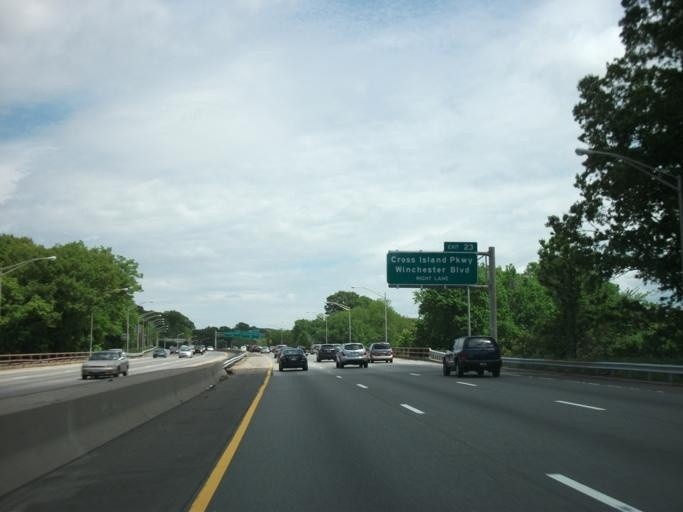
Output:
(233, 341), (393, 371)
(150, 343), (215, 360)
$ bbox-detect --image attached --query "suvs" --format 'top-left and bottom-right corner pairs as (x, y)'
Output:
(437, 336), (501, 378)
(81, 348), (127, 381)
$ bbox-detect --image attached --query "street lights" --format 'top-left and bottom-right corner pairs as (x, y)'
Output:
(273, 301), (352, 345)
(125, 299), (154, 353)
(572, 147), (681, 263)
(135, 312), (169, 352)
(350, 285), (389, 341)
(0, 253), (55, 278)
(86, 286), (130, 354)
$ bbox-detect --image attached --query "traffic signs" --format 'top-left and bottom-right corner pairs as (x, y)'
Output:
(441, 241), (479, 255)
(387, 250), (479, 287)
